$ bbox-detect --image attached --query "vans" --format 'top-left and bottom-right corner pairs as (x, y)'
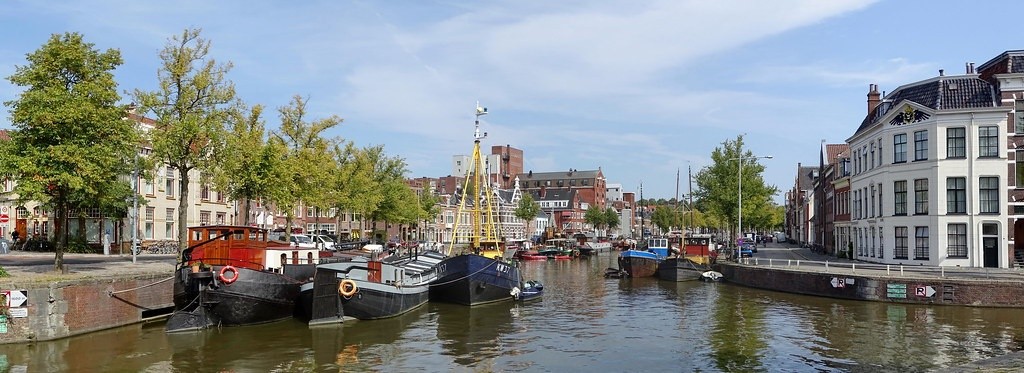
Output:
(303, 234), (342, 252)
(279, 232), (317, 249)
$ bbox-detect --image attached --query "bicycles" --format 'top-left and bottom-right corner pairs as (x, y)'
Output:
(11, 232), (53, 252)
(788, 237), (827, 256)
(128, 234), (178, 256)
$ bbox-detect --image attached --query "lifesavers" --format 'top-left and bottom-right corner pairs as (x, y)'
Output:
(575, 252), (579, 256)
(340, 279), (357, 296)
(219, 265), (239, 283)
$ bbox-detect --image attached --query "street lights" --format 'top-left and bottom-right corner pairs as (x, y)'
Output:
(728, 153), (775, 262)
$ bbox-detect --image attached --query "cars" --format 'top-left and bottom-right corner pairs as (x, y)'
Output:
(756, 234), (773, 248)
(742, 240), (757, 253)
(741, 237), (754, 242)
(736, 244), (753, 259)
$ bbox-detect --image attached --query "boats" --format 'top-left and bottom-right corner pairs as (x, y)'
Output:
(431, 98), (524, 306)
(506, 162), (725, 283)
(162, 224), (354, 334)
(515, 279), (544, 301)
(299, 237), (448, 320)
